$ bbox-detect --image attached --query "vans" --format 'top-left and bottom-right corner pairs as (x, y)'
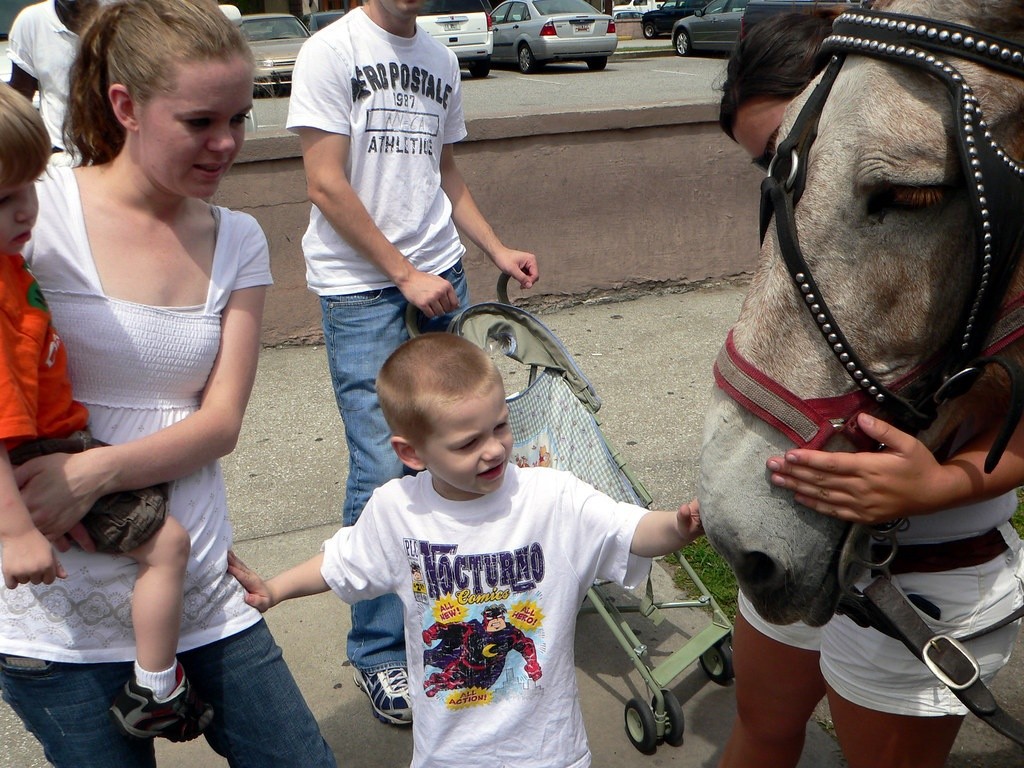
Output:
(417, 0), (494, 79)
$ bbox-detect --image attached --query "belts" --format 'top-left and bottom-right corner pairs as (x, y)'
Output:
(870, 520), (1015, 576)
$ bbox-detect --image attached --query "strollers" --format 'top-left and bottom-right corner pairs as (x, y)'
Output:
(405, 267), (738, 755)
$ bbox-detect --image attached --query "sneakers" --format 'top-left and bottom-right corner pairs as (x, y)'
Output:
(109, 662), (215, 742)
(354, 667), (413, 725)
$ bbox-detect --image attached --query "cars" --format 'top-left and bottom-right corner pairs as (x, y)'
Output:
(670, 0), (754, 57)
(600, 0), (685, 19)
(239, 11), (314, 97)
(488, 0), (619, 74)
(297, 8), (349, 38)
(640, 0), (716, 39)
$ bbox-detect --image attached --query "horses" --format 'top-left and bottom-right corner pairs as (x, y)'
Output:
(694, 0), (1024, 629)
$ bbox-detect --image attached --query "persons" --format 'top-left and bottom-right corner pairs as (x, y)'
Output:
(0, 0), (338, 768)
(285, 0), (541, 727)
(715, 0), (1024, 768)
(0, 83), (216, 742)
(6, 0), (120, 152)
(228, 332), (705, 768)
